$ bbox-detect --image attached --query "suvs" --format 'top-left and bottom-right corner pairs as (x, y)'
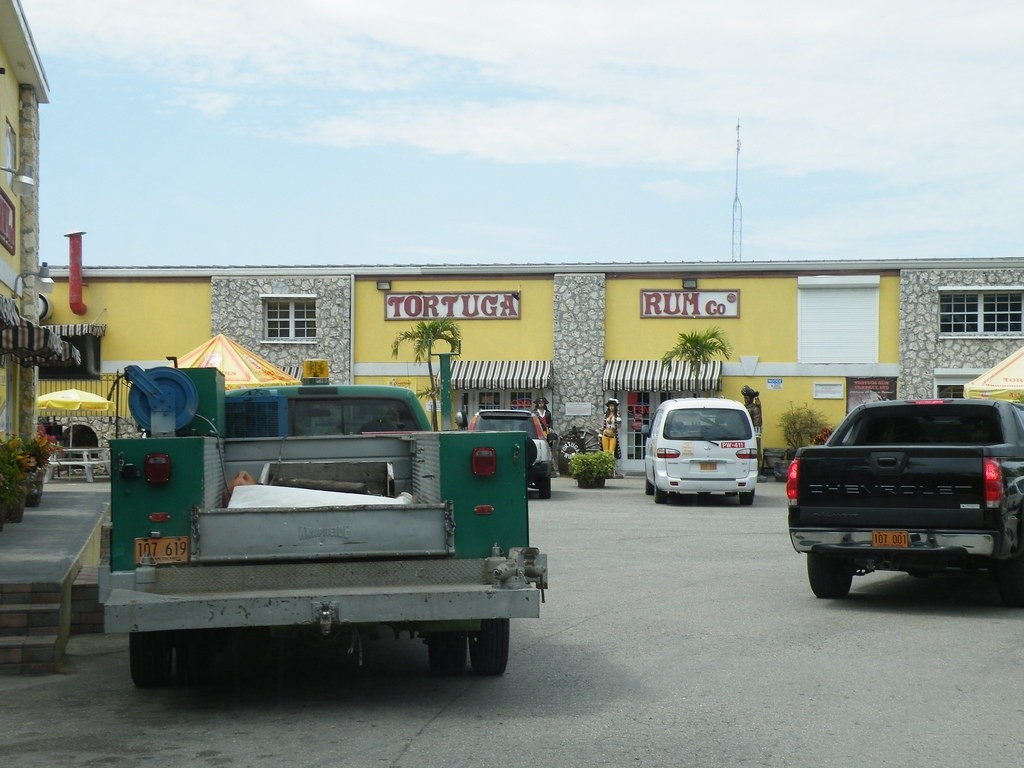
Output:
(467, 406), (554, 498)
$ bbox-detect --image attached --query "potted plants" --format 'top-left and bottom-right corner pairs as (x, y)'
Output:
(568, 450), (619, 488)
(783, 404), (823, 460)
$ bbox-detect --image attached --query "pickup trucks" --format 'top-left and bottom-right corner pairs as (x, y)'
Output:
(786, 397), (1024, 598)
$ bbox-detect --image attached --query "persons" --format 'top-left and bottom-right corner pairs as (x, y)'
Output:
(602, 398), (622, 478)
(740, 385), (763, 460)
(532, 398), (552, 440)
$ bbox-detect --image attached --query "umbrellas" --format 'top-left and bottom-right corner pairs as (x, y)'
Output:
(35, 388), (115, 479)
(164, 334), (302, 391)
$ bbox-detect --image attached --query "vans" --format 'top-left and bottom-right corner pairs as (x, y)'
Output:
(645, 397), (761, 503)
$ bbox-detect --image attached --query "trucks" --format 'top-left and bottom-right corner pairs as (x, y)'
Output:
(104, 359), (547, 677)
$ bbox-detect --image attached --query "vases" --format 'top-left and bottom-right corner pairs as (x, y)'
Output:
(7, 490), (25, 524)
(25, 480), (43, 506)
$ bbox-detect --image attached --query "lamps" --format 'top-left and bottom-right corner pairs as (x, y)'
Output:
(0, 167), (35, 197)
(14, 261), (54, 297)
(682, 279), (697, 288)
(376, 280), (391, 290)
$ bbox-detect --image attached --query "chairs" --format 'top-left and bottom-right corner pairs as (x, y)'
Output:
(358, 419), (395, 433)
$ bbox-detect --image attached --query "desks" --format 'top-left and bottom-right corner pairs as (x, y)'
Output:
(44, 447), (110, 484)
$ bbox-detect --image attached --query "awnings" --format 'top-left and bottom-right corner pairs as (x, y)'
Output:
(436, 360), (550, 390)
(0, 294), (81, 368)
(602, 360), (722, 393)
(40, 323), (107, 336)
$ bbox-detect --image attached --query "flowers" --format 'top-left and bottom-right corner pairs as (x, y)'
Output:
(0, 424), (65, 533)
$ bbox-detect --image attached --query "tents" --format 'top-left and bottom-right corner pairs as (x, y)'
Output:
(962, 346), (1024, 403)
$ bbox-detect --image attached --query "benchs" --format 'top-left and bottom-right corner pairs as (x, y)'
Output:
(43, 457), (111, 482)
